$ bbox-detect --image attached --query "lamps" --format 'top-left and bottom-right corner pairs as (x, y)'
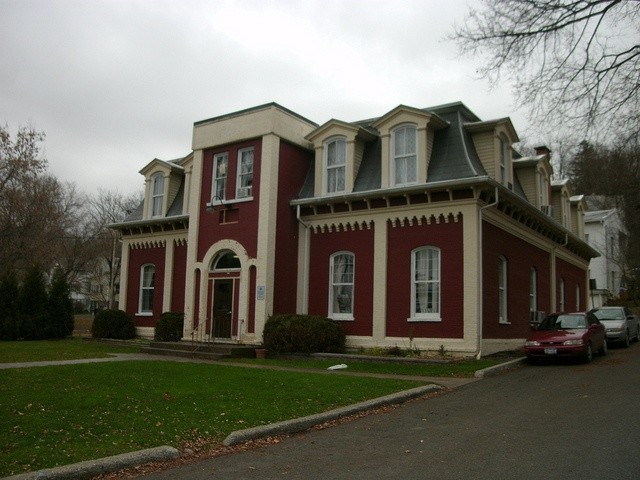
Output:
(206, 196), (231, 214)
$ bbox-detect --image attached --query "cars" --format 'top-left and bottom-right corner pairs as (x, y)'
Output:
(589, 305), (639, 348)
(522, 311), (608, 366)
